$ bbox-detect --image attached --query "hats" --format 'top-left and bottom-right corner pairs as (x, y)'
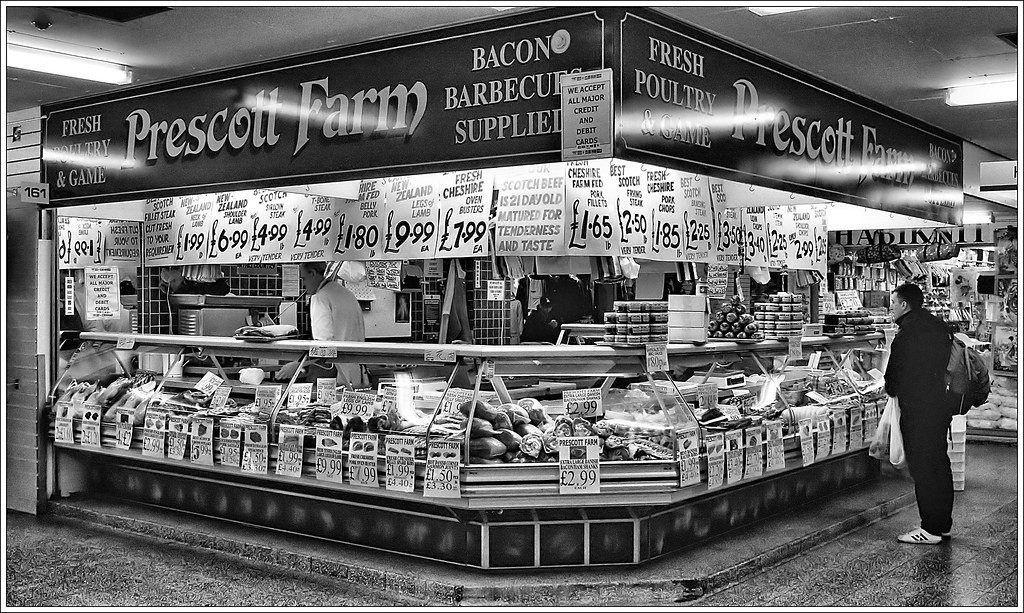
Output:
(540, 296), (552, 308)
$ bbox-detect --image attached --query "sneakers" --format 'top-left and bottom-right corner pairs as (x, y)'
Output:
(898, 527), (943, 544)
(942, 532), (951, 536)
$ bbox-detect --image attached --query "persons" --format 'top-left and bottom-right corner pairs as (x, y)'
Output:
(883, 284), (955, 545)
(276, 261), (370, 387)
(520, 295), (557, 342)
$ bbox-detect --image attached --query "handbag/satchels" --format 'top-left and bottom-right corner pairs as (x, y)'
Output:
(857, 233), (901, 263)
(916, 229), (960, 263)
(869, 395), (907, 469)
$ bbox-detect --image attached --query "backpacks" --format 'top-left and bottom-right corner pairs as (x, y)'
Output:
(943, 321), (990, 415)
